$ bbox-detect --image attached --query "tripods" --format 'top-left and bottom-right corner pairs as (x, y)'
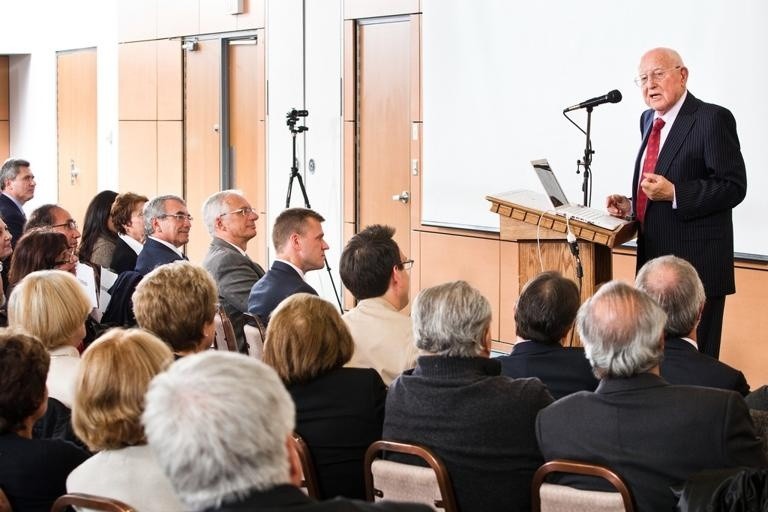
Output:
(285, 118), (344, 315)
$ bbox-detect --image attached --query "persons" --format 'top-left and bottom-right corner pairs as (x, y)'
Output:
(0, 156), (768, 511)
(606, 47), (748, 361)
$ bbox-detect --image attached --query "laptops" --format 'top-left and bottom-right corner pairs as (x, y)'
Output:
(529, 158), (628, 231)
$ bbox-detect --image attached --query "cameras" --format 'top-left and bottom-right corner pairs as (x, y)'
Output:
(286, 108), (309, 119)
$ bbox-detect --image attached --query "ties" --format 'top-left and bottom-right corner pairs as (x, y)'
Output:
(634, 116), (667, 226)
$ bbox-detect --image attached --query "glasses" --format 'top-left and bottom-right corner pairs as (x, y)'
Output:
(157, 214), (194, 223)
(397, 258), (415, 270)
(54, 245), (75, 266)
(634, 65), (682, 88)
(52, 220), (79, 230)
(220, 207), (257, 217)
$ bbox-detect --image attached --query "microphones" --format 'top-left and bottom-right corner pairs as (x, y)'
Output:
(563, 89), (622, 114)
